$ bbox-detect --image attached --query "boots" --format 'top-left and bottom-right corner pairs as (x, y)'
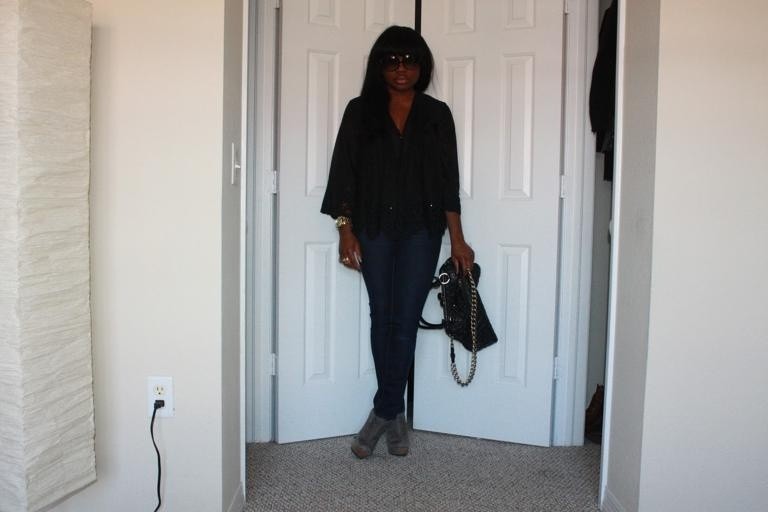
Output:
(352, 409), (392, 458)
(387, 412), (409, 456)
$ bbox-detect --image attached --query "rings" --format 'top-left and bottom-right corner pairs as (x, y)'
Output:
(342, 257), (350, 263)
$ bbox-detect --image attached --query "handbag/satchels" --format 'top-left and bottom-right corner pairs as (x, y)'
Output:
(419, 258), (497, 352)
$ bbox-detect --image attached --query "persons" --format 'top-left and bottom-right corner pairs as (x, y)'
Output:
(319, 24), (474, 458)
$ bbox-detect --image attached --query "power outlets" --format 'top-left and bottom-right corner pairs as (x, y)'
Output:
(147, 377), (177, 411)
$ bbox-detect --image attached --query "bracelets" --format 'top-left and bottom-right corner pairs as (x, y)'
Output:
(334, 215), (353, 229)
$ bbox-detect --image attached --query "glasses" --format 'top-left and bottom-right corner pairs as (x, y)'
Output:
(380, 55), (419, 72)
